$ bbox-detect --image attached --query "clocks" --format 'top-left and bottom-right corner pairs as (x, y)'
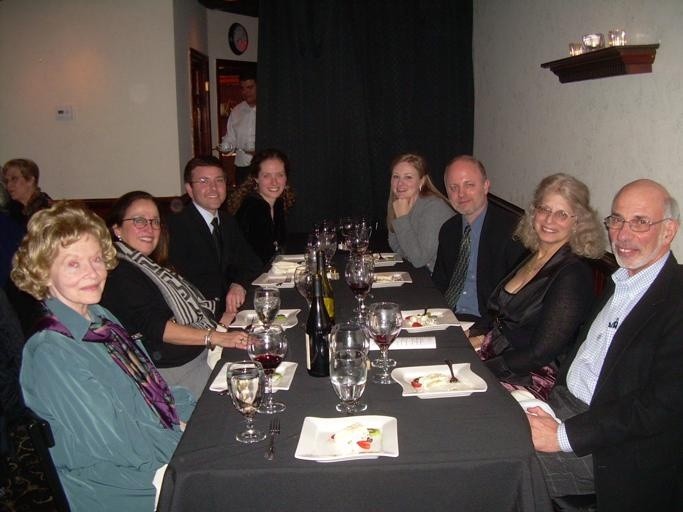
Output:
(228, 23), (249, 56)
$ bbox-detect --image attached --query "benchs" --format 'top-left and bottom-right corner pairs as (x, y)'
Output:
(437, 179), (683, 512)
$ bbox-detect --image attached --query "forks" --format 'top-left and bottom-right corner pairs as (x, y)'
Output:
(266, 418), (282, 462)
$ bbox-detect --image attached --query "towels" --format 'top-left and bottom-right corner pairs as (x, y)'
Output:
(509, 388), (562, 425)
(151, 463), (169, 508)
(207, 323), (227, 370)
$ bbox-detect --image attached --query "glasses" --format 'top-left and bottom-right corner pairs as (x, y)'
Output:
(3, 175), (27, 186)
(533, 204), (578, 222)
(114, 217), (161, 231)
(604, 214), (670, 232)
(189, 176), (226, 186)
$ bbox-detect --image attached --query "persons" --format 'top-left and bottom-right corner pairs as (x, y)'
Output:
(99, 191), (254, 431)
(459, 172), (606, 400)
(386, 154), (456, 272)
(432, 158), (532, 337)
(0, 157), (53, 487)
(229, 148), (296, 273)
(9, 199), (198, 512)
(221, 71), (256, 185)
(506, 178), (683, 512)
(169, 154), (261, 328)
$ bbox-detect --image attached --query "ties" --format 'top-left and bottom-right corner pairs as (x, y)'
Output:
(210, 217), (223, 256)
(443, 224), (471, 312)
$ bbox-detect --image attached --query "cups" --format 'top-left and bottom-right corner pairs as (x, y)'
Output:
(570, 28), (627, 57)
(246, 142), (255, 150)
(220, 143), (232, 152)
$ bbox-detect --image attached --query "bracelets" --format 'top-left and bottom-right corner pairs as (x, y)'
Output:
(205, 329), (216, 351)
(229, 282), (248, 296)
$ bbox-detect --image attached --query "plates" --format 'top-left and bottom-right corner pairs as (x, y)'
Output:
(359, 251), (416, 289)
(229, 308), (300, 331)
(207, 360), (298, 394)
(398, 308), (462, 334)
(239, 148), (256, 153)
(292, 415), (403, 463)
(250, 254), (309, 288)
(390, 360), (486, 398)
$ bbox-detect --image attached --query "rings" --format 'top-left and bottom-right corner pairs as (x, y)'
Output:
(241, 338), (244, 343)
(235, 343), (237, 348)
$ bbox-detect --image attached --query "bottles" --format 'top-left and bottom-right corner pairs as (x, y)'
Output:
(305, 274), (333, 380)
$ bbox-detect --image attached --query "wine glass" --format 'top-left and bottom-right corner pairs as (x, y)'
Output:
(326, 304), (404, 416)
(295, 215), (373, 322)
(226, 286), (288, 444)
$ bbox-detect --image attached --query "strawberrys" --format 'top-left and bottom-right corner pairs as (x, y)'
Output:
(357, 441), (369, 449)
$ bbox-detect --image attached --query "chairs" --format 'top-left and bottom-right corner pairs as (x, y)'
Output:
(226, 174), (294, 216)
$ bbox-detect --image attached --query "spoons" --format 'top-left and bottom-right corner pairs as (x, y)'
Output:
(444, 356), (458, 385)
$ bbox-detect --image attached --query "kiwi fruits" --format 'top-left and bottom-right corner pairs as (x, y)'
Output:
(367, 428), (379, 436)
